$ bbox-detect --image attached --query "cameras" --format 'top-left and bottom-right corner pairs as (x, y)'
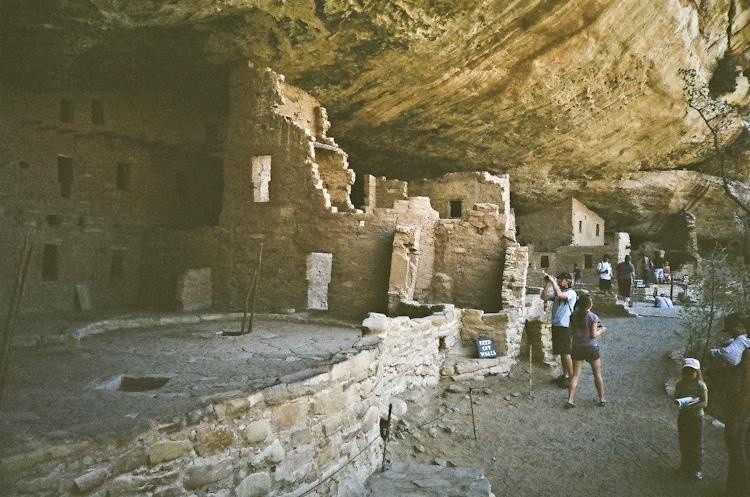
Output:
(544, 275), (548, 281)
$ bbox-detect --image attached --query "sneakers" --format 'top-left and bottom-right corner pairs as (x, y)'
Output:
(675, 467), (704, 481)
(552, 375), (569, 389)
(565, 401), (576, 408)
(600, 398), (608, 407)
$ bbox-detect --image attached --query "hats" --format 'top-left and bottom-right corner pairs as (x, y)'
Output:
(556, 271), (572, 279)
(721, 310), (745, 333)
(681, 357), (700, 370)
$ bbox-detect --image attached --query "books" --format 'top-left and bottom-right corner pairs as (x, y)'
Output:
(675, 396), (700, 409)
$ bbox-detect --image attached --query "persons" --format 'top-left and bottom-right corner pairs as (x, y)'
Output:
(597, 251), (674, 309)
(566, 294), (608, 408)
(573, 263), (582, 283)
(540, 272), (576, 386)
(704, 311), (750, 496)
(672, 357), (710, 480)
